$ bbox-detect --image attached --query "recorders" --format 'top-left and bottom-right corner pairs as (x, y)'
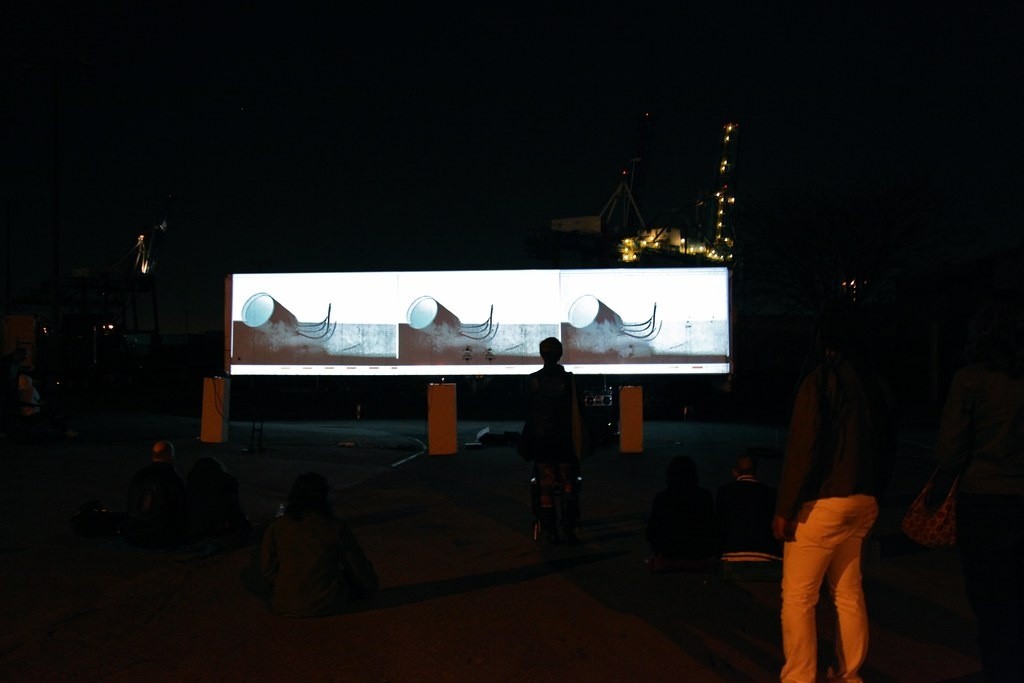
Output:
(581, 386), (613, 407)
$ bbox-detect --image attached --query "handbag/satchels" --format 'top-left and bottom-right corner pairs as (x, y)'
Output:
(901, 463), (959, 548)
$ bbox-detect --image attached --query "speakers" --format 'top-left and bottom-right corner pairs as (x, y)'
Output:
(581, 404), (616, 449)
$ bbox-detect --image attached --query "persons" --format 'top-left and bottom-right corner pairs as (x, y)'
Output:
(254, 471), (373, 618)
(645, 455), (715, 560)
(714, 446), (784, 560)
(116, 441), (256, 557)
(773, 297), (898, 683)
(934, 288), (1023, 682)
(516, 338), (596, 546)
(0, 348), (79, 440)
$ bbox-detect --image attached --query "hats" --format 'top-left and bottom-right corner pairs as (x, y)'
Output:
(152, 441), (174, 462)
